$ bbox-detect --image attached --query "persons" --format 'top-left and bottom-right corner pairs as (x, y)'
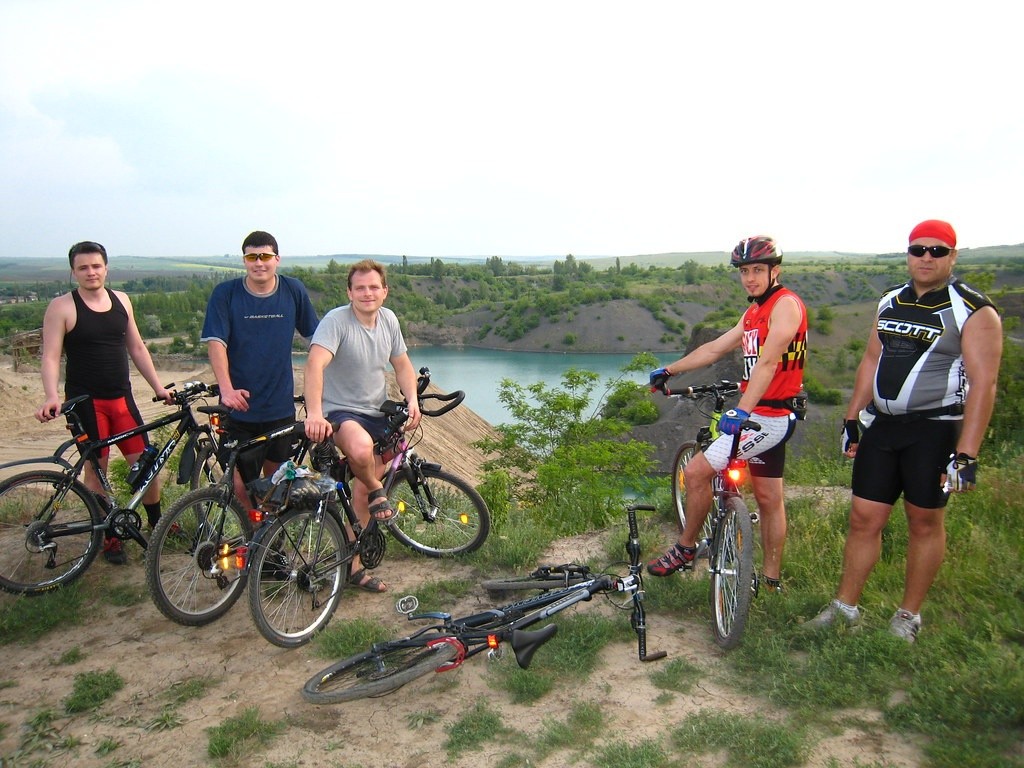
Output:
(201, 231), (321, 583)
(34, 241), (184, 564)
(799, 221), (1003, 646)
(647, 236), (809, 591)
(304, 259), (422, 594)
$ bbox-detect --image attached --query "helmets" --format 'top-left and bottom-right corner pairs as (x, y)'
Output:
(729, 236), (784, 268)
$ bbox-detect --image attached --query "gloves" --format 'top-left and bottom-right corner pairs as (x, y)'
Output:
(650, 366), (671, 394)
(715, 408), (751, 436)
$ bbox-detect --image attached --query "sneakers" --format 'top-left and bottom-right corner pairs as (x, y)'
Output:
(888, 608), (921, 645)
(102, 533), (127, 565)
(147, 520), (184, 537)
(646, 541), (696, 577)
(800, 600), (863, 636)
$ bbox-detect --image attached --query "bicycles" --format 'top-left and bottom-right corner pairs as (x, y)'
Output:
(298, 506), (668, 703)
(242, 367), (493, 649)
(144, 395), (326, 627)
(656, 376), (759, 650)
(0, 380), (240, 597)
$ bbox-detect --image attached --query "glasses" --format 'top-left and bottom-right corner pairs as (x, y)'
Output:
(242, 253), (277, 262)
(908, 245), (950, 259)
(71, 243), (101, 252)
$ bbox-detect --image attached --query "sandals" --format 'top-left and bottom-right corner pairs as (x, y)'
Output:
(368, 488), (395, 522)
(347, 569), (388, 593)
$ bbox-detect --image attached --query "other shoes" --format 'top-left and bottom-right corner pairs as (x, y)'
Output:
(260, 550), (292, 579)
(758, 575), (789, 599)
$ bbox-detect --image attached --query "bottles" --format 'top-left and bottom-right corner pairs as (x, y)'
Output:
(313, 475), (343, 494)
(125, 442), (160, 489)
(256, 477), (274, 490)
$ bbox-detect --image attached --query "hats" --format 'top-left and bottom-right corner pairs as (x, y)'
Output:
(908, 219), (957, 247)
